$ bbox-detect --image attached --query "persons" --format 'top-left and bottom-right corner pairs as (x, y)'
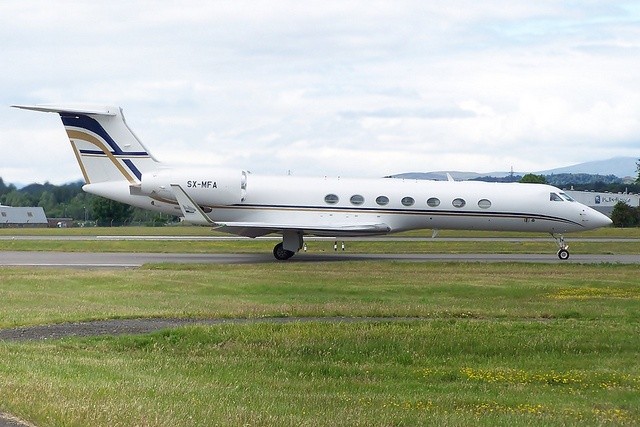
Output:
(341, 241), (345, 251)
(304, 242), (308, 252)
(334, 241), (338, 252)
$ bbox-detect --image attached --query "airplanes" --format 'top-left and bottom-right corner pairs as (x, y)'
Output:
(10, 104), (614, 260)
